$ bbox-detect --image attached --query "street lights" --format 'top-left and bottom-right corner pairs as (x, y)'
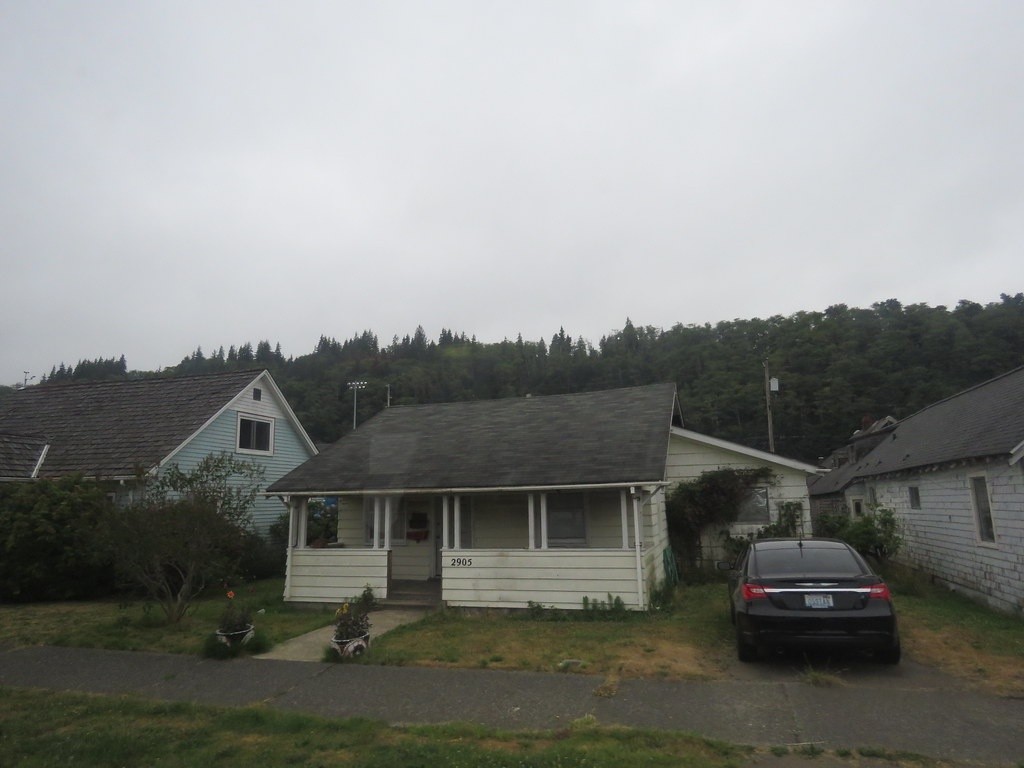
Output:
(347, 381), (367, 430)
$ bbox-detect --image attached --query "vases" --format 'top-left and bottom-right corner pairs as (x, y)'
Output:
(214, 625), (256, 649)
(332, 629), (374, 658)
(315, 536), (328, 548)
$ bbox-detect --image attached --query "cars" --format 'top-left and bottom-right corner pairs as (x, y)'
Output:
(717, 532), (901, 665)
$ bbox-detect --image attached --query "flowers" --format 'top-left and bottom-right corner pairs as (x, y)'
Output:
(313, 511), (332, 538)
(334, 583), (381, 639)
(219, 584), (253, 632)
